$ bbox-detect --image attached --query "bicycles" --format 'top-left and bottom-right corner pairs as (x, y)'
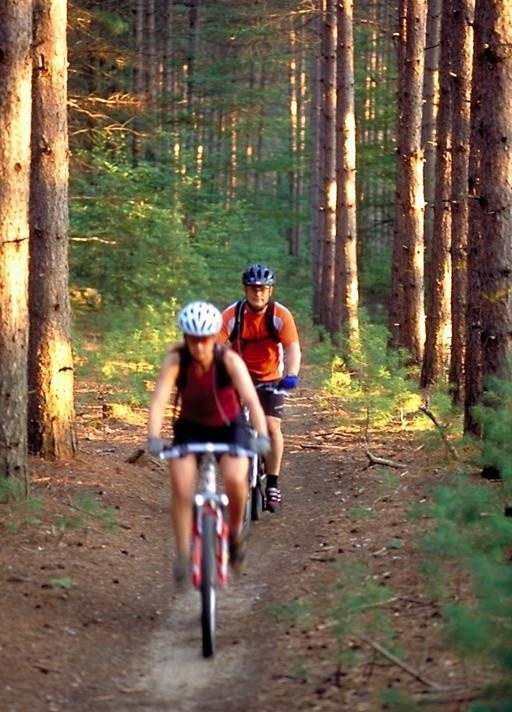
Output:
(242, 377), (293, 523)
(154, 440), (263, 661)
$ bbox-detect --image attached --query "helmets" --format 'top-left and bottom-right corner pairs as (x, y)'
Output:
(241, 264), (276, 286)
(177, 301), (223, 337)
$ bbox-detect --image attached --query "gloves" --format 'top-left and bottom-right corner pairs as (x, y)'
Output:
(251, 433), (272, 455)
(282, 373), (297, 390)
(146, 437), (170, 458)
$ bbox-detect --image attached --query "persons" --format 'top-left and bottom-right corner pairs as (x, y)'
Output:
(146, 300), (269, 594)
(217, 266), (303, 511)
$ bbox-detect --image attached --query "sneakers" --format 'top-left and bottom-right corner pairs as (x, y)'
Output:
(172, 557), (193, 595)
(227, 532), (248, 575)
(265, 487), (282, 514)
